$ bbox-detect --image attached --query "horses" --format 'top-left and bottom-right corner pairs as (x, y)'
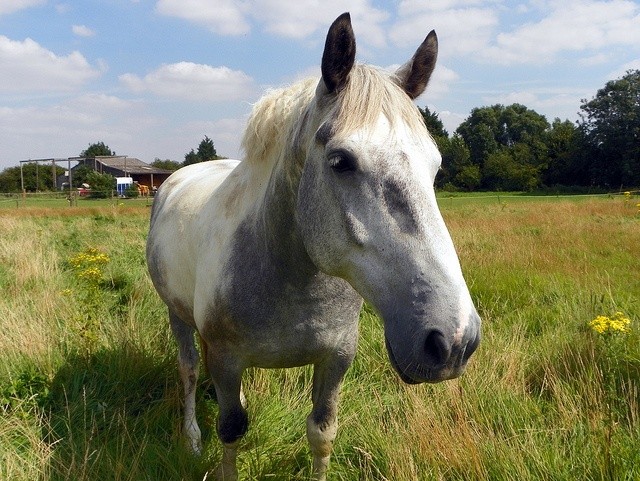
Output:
(146, 11), (482, 481)
